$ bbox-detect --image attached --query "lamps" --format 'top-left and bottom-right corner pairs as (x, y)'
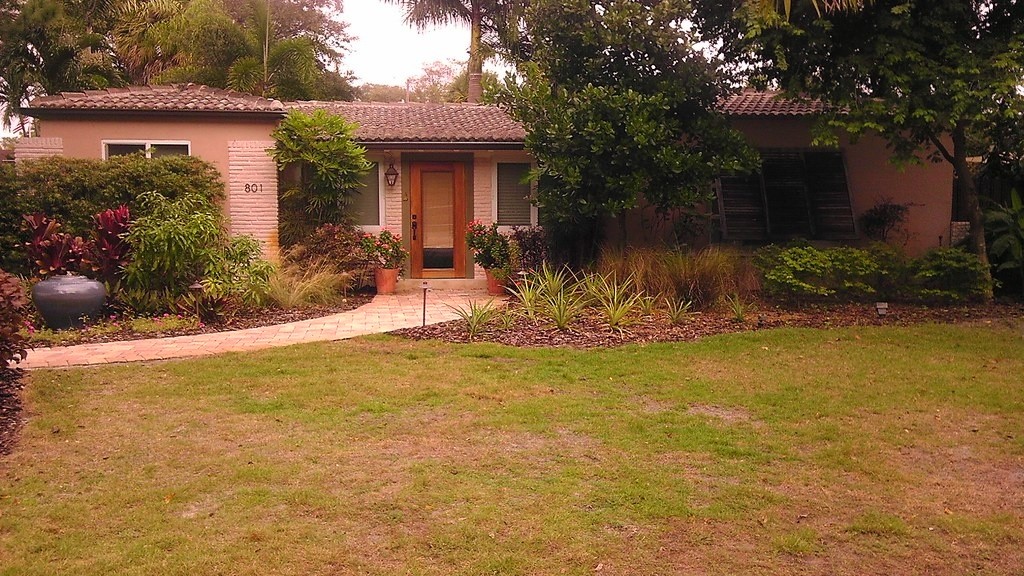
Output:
(385, 163), (398, 186)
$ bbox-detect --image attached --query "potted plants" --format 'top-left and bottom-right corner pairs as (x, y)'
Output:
(360, 226), (409, 267)
(464, 218), (513, 269)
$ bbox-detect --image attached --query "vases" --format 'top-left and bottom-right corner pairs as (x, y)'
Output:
(485, 266), (509, 296)
(375, 267), (403, 294)
